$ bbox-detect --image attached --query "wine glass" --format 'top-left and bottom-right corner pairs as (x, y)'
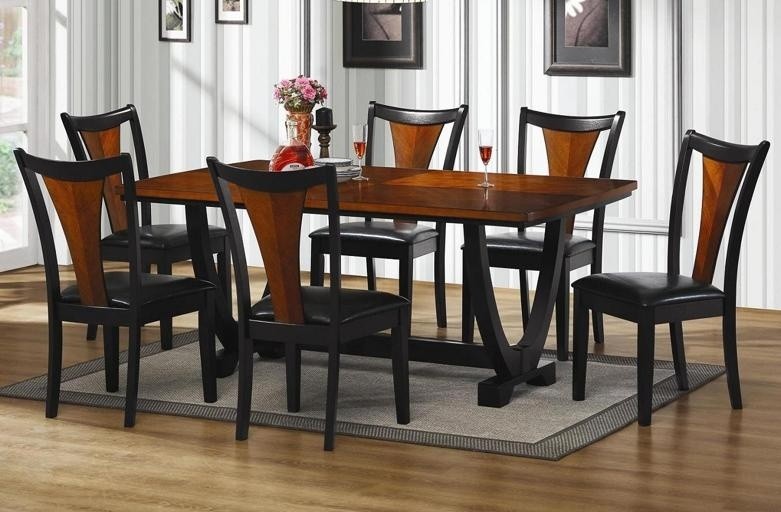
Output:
(351, 124), (373, 180)
(475, 130), (497, 190)
(482, 191), (494, 211)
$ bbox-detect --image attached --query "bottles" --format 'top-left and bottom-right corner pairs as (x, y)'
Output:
(268, 120), (314, 174)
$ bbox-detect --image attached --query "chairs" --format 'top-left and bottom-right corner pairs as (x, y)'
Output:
(308, 99), (469, 331)
(205, 152), (414, 455)
(10, 146), (221, 431)
(571, 129), (771, 430)
(62, 102), (236, 352)
(458, 106), (628, 364)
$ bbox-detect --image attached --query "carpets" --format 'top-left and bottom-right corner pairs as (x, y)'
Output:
(0, 320), (729, 463)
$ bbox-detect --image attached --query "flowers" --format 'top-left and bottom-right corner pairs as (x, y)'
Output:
(272, 75), (328, 109)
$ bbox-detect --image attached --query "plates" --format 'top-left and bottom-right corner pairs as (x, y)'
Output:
(314, 157), (360, 184)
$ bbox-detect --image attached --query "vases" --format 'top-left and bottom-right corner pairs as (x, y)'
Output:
(286, 101), (315, 154)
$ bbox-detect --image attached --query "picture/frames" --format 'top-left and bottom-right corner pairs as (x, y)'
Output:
(341, 2), (423, 70)
(215, 0), (248, 25)
(158, 0), (193, 43)
(543, 1), (632, 78)
